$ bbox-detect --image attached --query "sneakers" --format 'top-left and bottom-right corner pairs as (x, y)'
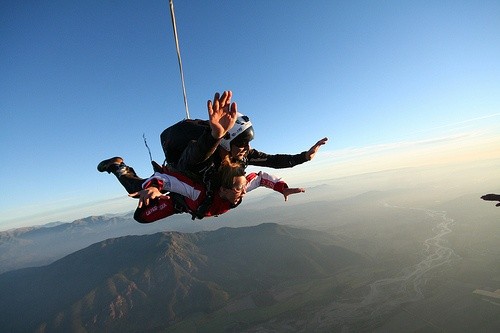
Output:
(97, 157), (123, 173)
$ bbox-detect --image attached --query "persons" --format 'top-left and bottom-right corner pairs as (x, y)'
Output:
(127, 169), (306, 223)
(97, 90), (328, 198)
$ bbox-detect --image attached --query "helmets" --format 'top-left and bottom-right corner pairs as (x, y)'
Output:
(220, 112), (252, 151)
